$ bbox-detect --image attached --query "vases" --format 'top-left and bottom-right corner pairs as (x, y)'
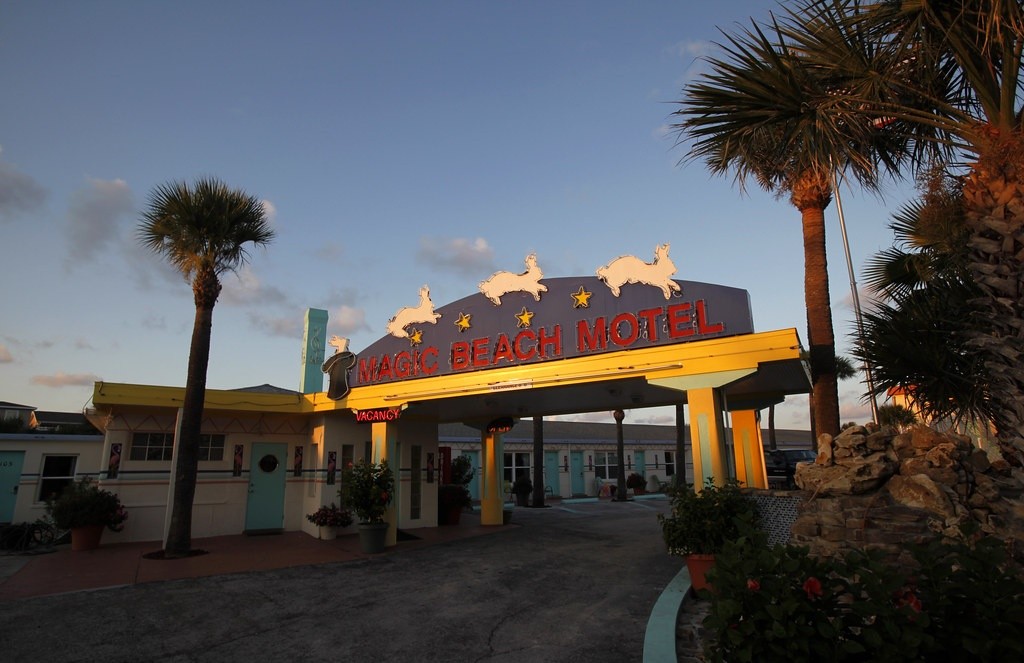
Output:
(71, 525), (105, 549)
(320, 525), (338, 541)
(503, 509), (514, 525)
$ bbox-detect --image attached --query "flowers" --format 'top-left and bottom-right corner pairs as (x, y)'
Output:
(306, 502), (354, 529)
(45, 475), (129, 534)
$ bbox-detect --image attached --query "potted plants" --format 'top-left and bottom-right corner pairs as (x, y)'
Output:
(335, 457), (396, 553)
(657, 475), (764, 599)
(438, 456), (476, 527)
(511, 475), (533, 507)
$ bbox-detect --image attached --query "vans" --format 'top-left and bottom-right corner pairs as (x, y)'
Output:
(762, 447), (818, 492)
